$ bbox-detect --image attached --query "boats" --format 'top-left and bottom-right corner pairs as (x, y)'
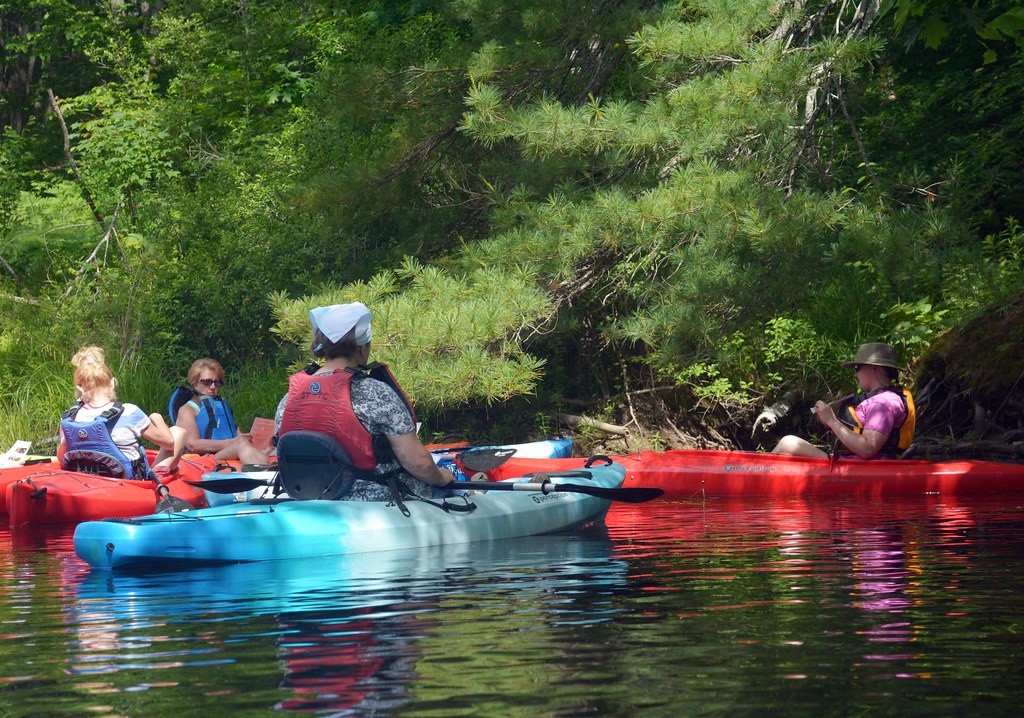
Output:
(455, 448), (1024, 498)
(2, 429), (576, 528)
(73, 457), (625, 576)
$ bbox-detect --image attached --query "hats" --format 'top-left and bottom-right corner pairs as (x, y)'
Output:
(842, 342), (906, 372)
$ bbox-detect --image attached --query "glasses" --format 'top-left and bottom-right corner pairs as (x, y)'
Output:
(855, 364), (866, 372)
(200, 378), (224, 386)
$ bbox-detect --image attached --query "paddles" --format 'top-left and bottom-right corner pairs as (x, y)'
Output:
(147, 466), (196, 515)
(185, 476), (662, 503)
(240, 448), (519, 474)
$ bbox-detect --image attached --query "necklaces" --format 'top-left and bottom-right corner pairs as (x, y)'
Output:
(87, 400), (111, 408)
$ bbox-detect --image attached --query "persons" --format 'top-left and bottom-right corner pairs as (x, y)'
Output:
(175, 358), (269, 467)
(56, 347), (188, 482)
(769, 341), (915, 461)
(274, 300), (476, 502)
